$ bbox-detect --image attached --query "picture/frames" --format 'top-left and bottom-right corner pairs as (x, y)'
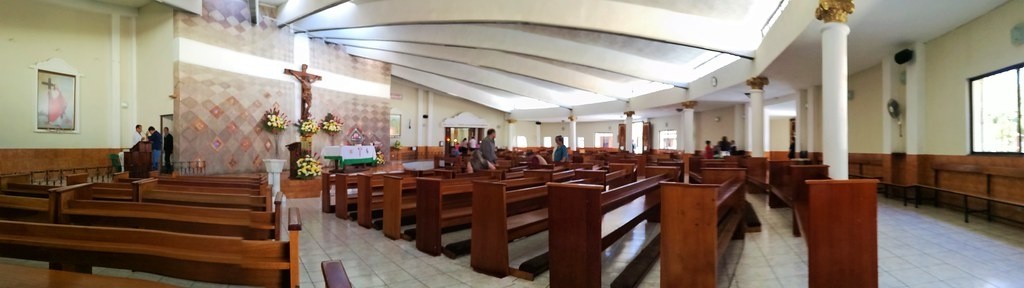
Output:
(30, 57), (84, 134)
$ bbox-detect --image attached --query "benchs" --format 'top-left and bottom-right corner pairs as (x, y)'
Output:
(0, 147), (883, 288)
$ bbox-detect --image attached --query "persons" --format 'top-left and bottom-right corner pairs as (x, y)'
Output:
(699, 135), (737, 157)
(133, 125), (145, 146)
(290, 70), (318, 119)
(146, 126), (162, 171)
(163, 127), (173, 174)
(787, 137), (795, 159)
(552, 134), (568, 162)
(479, 128), (498, 167)
(461, 138), (467, 161)
(466, 150), (496, 174)
(525, 150), (547, 165)
(469, 136), (477, 152)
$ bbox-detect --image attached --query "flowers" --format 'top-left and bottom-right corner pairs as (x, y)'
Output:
(374, 145), (386, 165)
(260, 107), (291, 134)
(320, 113), (344, 137)
(294, 150), (322, 176)
(294, 116), (319, 139)
